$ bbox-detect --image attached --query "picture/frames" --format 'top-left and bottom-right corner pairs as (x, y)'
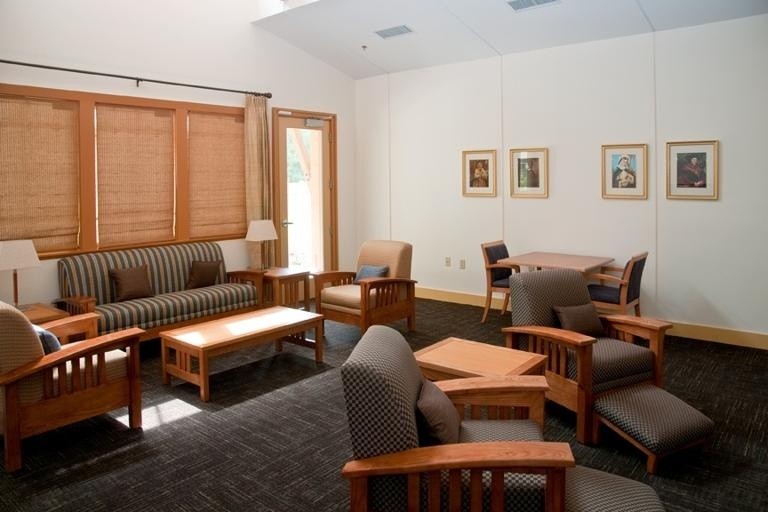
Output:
(600, 143), (648, 200)
(508, 147), (549, 199)
(664, 140), (719, 201)
(462, 149), (497, 197)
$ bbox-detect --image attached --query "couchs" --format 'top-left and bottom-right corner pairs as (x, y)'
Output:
(50, 241), (268, 351)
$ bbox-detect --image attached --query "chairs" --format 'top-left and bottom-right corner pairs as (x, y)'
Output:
(480, 240), (520, 324)
(587, 251), (648, 340)
(0, 300), (147, 472)
(313, 241), (417, 339)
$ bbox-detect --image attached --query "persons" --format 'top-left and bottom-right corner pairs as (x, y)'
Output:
(677, 153), (706, 187)
(472, 162), (487, 187)
(612, 154), (636, 188)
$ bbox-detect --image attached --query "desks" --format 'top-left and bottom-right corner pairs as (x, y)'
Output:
(496, 251), (615, 274)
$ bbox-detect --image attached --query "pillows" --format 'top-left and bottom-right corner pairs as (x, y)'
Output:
(30, 323), (61, 354)
(108, 263), (154, 301)
(186, 261), (221, 289)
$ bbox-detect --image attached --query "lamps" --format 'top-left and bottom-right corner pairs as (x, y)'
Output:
(0, 239), (42, 313)
(244, 218), (279, 273)
